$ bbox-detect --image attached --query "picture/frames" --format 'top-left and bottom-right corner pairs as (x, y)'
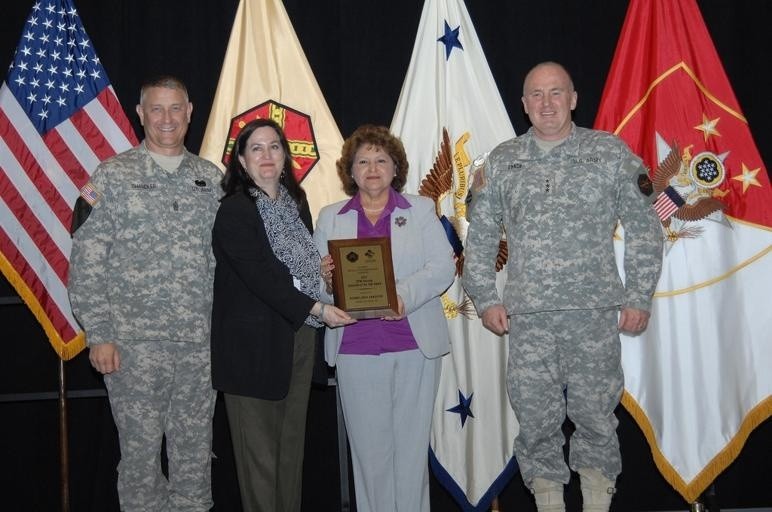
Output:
(328, 237), (400, 321)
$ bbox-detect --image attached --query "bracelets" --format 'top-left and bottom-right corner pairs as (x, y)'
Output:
(312, 299), (329, 327)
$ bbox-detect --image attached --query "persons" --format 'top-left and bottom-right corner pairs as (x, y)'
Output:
(462, 63), (666, 510)
(210, 121), (358, 509)
(62, 74), (228, 512)
(310, 125), (455, 510)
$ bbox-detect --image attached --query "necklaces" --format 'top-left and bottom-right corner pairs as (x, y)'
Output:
(363, 206), (385, 213)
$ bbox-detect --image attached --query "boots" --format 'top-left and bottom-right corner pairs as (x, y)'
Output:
(531, 477), (566, 511)
(576, 466), (617, 511)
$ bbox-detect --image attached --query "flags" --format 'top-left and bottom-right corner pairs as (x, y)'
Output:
(0, 3), (146, 361)
(373, 0), (528, 510)
(195, 2), (349, 228)
(593, 2), (769, 511)
